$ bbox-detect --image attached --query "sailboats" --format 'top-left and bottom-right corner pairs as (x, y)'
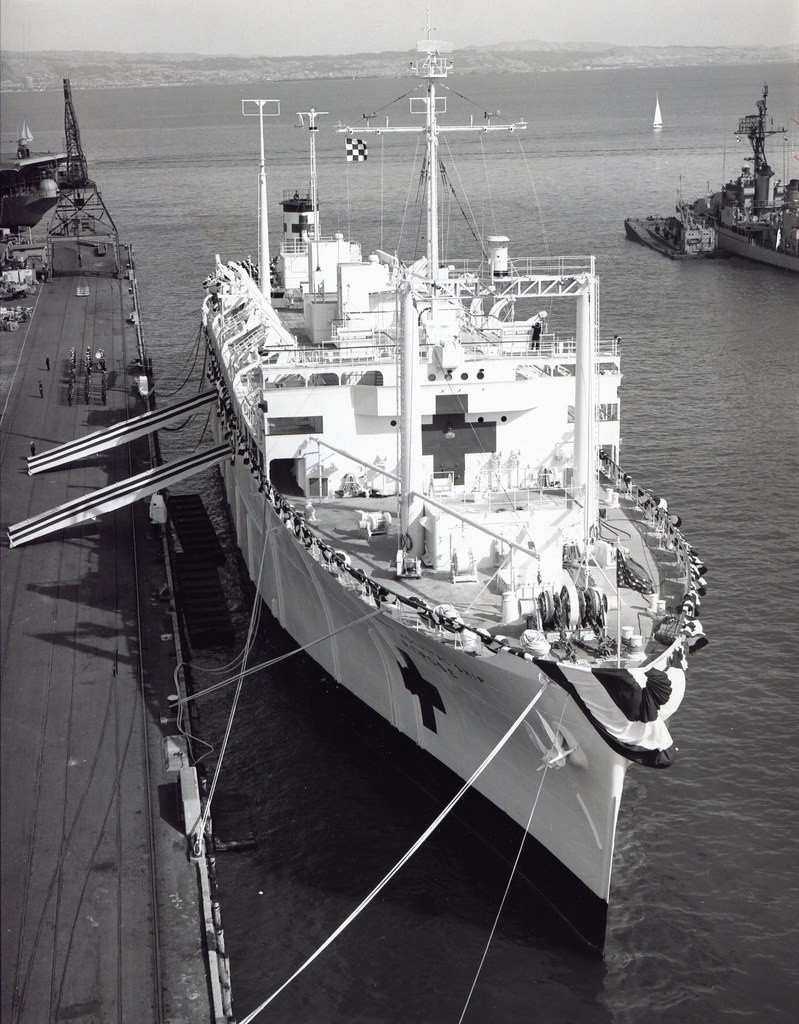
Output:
(651, 90), (663, 129)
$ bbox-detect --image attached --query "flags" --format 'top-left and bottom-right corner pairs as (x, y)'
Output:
(346, 137), (368, 162)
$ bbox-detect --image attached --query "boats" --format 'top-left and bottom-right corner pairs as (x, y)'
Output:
(201, 1), (708, 962)
(624, 174), (718, 260)
(676, 80), (799, 271)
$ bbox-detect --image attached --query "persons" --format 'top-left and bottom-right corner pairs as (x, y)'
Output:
(38, 380), (43, 398)
(30, 440), (35, 455)
(531, 321), (544, 351)
(68, 346), (107, 407)
(44, 354), (49, 371)
